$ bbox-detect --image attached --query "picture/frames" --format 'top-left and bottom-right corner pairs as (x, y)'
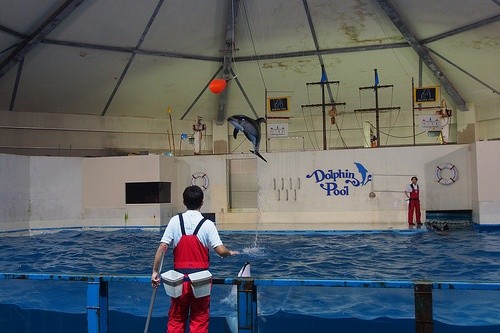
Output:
(414, 87), (439, 104)
(267, 96), (290, 112)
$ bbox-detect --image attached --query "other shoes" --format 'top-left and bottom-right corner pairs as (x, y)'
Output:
(408, 223), (415, 226)
(417, 221), (423, 226)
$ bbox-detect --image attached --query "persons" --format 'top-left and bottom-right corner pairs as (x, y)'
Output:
(404, 176), (424, 226)
(149, 186), (238, 333)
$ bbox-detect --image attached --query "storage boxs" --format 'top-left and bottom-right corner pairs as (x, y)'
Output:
(188, 270), (212, 297)
(160, 270), (184, 298)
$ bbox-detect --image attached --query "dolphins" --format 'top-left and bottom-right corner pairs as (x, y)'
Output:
(225, 262), (266, 333)
(227, 115), (267, 162)
